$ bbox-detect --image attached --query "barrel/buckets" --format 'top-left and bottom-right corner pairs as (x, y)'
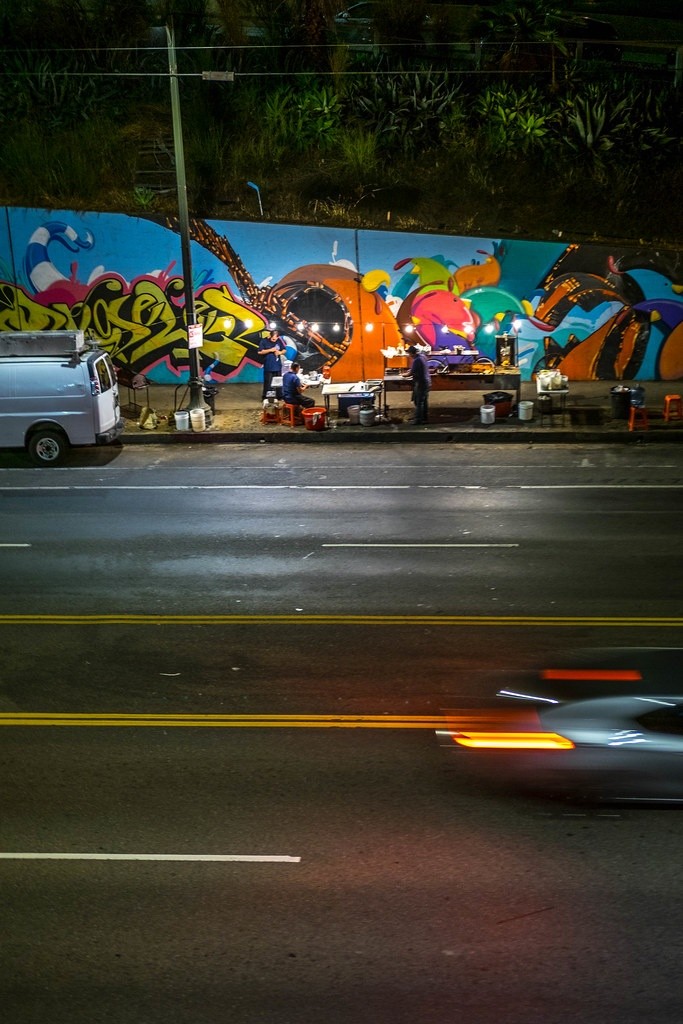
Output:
(302, 407), (326, 430)
(348, 405), (360, 424)
(481, 405), (496, 424)
(174, 411), (189, 430)
(519, 401), (534, 420)
(190, 409), (206, 432)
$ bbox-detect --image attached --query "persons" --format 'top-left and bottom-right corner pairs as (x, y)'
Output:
(401, 346), (432, 424)
(257, 330), (286, 403)
(283, 363), (315, 408)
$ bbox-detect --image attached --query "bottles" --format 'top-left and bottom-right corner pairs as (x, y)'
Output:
(630, 382), (645, 407)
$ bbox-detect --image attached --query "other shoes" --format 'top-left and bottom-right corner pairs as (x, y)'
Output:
(412, 419), (427, 425)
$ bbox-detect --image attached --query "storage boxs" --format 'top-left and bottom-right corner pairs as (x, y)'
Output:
(337, 394), (376, 417)
(365, 379), (382, 391)
(483, 392), (514, 417)
(610, 385), (644, 419)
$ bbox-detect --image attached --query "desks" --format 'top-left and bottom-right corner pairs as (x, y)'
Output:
(272, 377), (320, 402)
(322, 383), (382, 425)
(384, 366), (521, 419)
(536, 376), (569, 428)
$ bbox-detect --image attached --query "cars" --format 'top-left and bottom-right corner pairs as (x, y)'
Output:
(442, 694), (683, 808)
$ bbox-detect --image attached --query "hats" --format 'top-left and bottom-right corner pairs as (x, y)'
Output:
(405, 346), (420, 353)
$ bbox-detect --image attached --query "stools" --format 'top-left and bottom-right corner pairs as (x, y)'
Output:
(263, 399), (278, 423)
(628, 405), (649, 432)
(280, 403), (302, 429)
(663, 394), (683, 422)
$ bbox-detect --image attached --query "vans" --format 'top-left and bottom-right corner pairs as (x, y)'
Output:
(0, 330), (125, 467)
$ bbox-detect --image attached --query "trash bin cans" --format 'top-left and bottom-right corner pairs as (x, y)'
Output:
(483, 392), (513, 415)
(610, 385), (630, 419)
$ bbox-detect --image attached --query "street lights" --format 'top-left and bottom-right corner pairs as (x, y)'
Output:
(145, 3), (214, 426)
